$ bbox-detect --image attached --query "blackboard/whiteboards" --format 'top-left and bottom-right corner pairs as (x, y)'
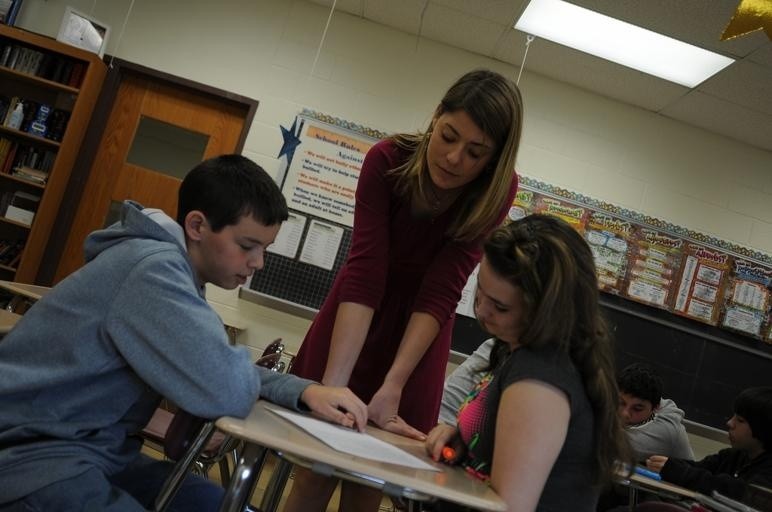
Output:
(238, 107), (772, 446)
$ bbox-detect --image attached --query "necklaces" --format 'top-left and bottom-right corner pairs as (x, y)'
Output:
(429, 184), (452, 213)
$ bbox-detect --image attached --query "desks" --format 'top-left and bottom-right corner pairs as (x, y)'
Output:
(1, 308), (24, 334)
(146, 403), (508, 512)
(0, 279), (55, 311)
(610, 465), (701, 511)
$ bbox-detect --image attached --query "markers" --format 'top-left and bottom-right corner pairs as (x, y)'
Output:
(443, 447), (455, 459)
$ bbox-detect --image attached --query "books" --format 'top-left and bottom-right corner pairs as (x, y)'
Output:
(1, 41), (83, 267)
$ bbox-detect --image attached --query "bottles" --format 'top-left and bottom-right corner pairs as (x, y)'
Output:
(26, 103), (54, 135)
(7, 102), (24, 131)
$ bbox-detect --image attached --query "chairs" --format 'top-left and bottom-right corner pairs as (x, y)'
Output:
(112, 416), (226, 510)
(141, 332), (284, 487)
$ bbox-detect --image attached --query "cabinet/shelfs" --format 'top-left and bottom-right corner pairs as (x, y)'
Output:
(0, 25), (110, 314)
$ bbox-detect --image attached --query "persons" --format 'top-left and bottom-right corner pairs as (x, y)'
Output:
(0, 151), (370, 511)
(635, 384), (772, 510)
(613, 359), (699, 512)
(280, 68), (524, 511)
(436, 336), (497, 428)
(427, 210), (639, 510)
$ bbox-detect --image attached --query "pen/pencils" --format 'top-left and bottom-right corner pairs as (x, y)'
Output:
(461, 462), (486, 481)
(613, 460), (662, 481)
